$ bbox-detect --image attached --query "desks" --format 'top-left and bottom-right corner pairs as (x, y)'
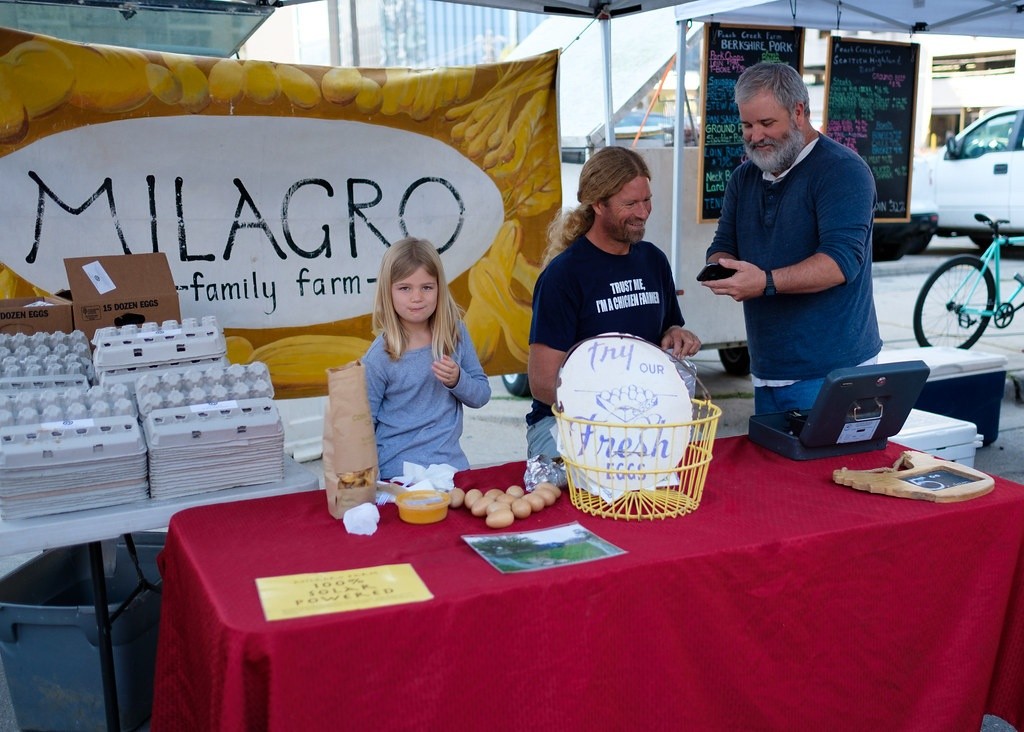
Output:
(146, 439), (1024, 732)
(0, 454), (320, 732)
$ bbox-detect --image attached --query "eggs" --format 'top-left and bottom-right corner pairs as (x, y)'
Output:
(448, 482), (561, 528)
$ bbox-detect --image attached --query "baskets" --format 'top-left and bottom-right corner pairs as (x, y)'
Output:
(550, 399), (722, 521)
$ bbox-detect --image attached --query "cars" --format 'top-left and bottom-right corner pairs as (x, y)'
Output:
(901, 106), (1024, 256)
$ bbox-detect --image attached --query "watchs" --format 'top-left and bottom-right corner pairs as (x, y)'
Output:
(762, 270), (776, 296)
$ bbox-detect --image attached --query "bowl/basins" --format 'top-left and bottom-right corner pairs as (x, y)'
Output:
(396, 490), (452, 525)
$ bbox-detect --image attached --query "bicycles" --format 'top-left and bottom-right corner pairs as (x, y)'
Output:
(912, 212), (1024, 355)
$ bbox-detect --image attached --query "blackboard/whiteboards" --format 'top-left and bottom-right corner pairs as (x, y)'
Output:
(698, 21), (806, 224)
(821, 35), (922, 224)
(895, 465), (977, 494)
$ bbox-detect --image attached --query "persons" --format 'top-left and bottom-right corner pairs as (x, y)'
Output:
(527, 145), (702, 458)
(706, 62), (884, 415)
(361, 237), (493, 480)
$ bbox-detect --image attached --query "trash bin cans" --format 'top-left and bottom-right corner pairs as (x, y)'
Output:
(0, 531), (169, 732)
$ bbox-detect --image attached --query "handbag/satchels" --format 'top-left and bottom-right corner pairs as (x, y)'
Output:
(325, 360), (379, 519)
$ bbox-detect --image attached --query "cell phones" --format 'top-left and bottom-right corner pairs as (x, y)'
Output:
(697, 263), (737, 281)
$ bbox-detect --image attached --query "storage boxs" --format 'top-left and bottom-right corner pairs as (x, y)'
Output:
(888, 407), (985, 469)
(862, 345), (1011, 449)
(745, 360), (930, 462)
(62, 252), (182, 350)
(0, 295), (73, 337)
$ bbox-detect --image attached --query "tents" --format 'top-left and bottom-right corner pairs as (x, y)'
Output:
(432, 0), (1023, 290)
